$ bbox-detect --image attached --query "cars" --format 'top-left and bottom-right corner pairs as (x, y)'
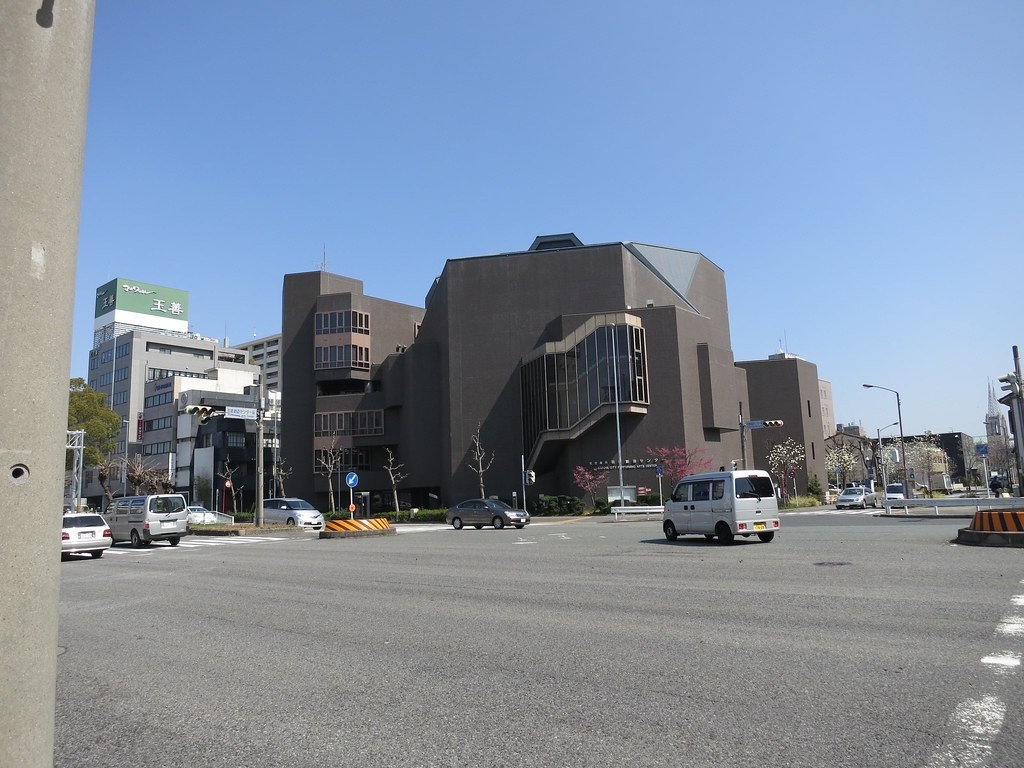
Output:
(187, 506), (216, 524)
(446, 498), (530, 529)
(836, 487), (877, 509)
(62, 513), (112, 560)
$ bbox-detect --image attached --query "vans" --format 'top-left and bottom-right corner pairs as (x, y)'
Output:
(881, 483), (906, 508)
(663, 470), (780, 545)
(253, 498), (326, 532)
(103, 494), (186, 548)
(63, 504), (90, 514)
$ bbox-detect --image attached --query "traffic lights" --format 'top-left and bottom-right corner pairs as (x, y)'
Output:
(526, 470), (535, 486)
(763, 420), (783, 428)
(909, 467), (915, 480)
(184, 405), (215, 425)
(729, 461), (738, 471)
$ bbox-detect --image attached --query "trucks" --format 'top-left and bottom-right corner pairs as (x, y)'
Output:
(930, 473), (955, 495)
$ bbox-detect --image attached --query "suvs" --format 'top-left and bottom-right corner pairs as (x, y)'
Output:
(915, 482), (928, 493)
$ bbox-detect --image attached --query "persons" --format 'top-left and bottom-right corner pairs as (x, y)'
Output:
(989, 475), (1003, 499)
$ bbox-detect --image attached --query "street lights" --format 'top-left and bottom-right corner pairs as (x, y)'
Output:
(984, 422), (1013, 491)
(270, 389), (278, 497)
(877, 422), (899, 513)
(863, 384), (910, 508)
(610, 322), (624, 518)
